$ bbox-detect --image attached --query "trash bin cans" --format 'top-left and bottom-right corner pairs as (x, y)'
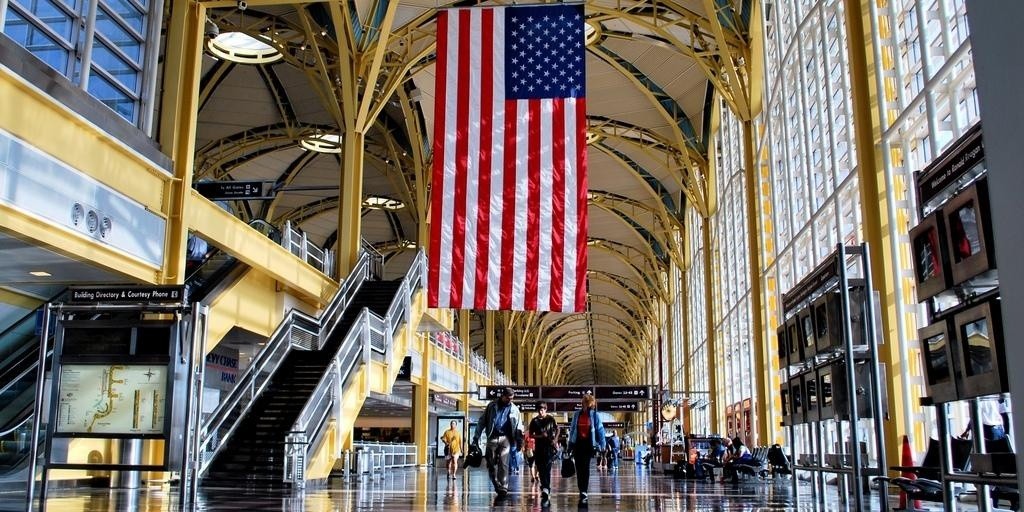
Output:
(120, 439), (143, 488)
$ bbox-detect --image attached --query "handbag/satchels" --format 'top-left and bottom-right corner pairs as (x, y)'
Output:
(445, 445), (451, 455)
(463, 438), (483, 470)
(561, 451), (577, 479)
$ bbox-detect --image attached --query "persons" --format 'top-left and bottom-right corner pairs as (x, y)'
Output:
(440, 418), (464, 482)
(522, 421), (631, 481)
(700, 438), (727, 484)
(598, 468), (610, 499)
(961, 394), (1011, 440)
(526, 480), (541, 512)
(528, 399), (561, 512)
(565, 394), (607, 511)
(442, 477), (460, 512)
(469, 386), (523, 505)
(719, 435), (737, 484)
(610, 467), (621, 511)
(722, 437), (752, 485)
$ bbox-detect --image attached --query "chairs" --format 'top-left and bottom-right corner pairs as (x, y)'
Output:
(703, 444), (792, 483)
(871, 437), (973, 511)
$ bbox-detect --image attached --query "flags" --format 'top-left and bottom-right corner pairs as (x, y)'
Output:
(423, 3), (588, 317)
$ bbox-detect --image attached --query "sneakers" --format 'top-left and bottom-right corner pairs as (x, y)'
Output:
(447, 474), (451, 479)
(541, 488), (550, 499)
(532, 475), (540, 482)
(452, 475), (457, 479)
(498, 486), (509, 496)
(579, 492), (589, 503)
(597, 465), (619, 470)
